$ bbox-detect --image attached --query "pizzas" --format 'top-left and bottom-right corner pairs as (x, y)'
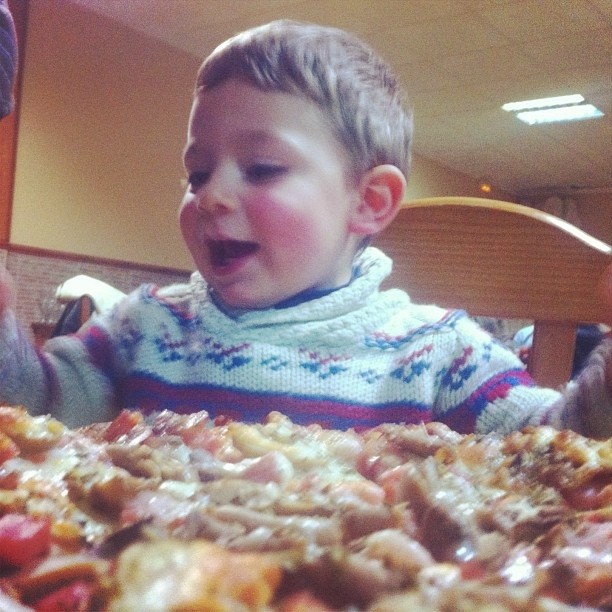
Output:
(1, 402), (611, 612)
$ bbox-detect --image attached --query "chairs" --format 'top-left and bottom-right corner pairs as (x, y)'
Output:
(354, 196), (612, 394)
(62, 296), (91, 338)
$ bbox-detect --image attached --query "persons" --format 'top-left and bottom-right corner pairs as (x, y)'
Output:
(2, 17), (611, 441)
(0, 1), (22, 120)
